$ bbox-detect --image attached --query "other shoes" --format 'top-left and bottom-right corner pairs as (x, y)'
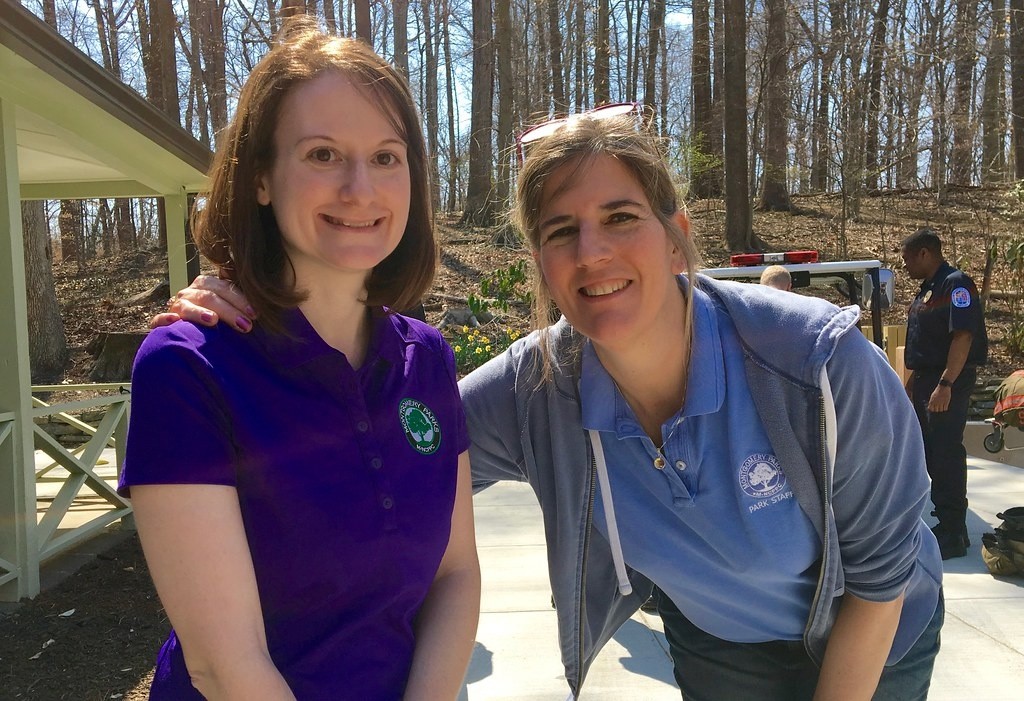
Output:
(929, 524), (970, 560)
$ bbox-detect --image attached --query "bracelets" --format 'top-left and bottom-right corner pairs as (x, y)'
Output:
(938, 379), (954, 388)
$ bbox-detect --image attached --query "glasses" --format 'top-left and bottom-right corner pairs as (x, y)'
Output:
(515, 102), (657, 194)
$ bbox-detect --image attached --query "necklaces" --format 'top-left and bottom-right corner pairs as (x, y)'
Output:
(613, 280), (690, 468)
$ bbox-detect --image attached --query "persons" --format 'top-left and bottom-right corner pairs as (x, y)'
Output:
(150, 103), (949, 700)
(905, 229), (989, 560)
(760, 264), (793, 291)
(120, 10), (483, 701)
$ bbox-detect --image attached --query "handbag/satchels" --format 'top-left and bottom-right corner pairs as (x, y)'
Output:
(981, 506), (1024, 575)
